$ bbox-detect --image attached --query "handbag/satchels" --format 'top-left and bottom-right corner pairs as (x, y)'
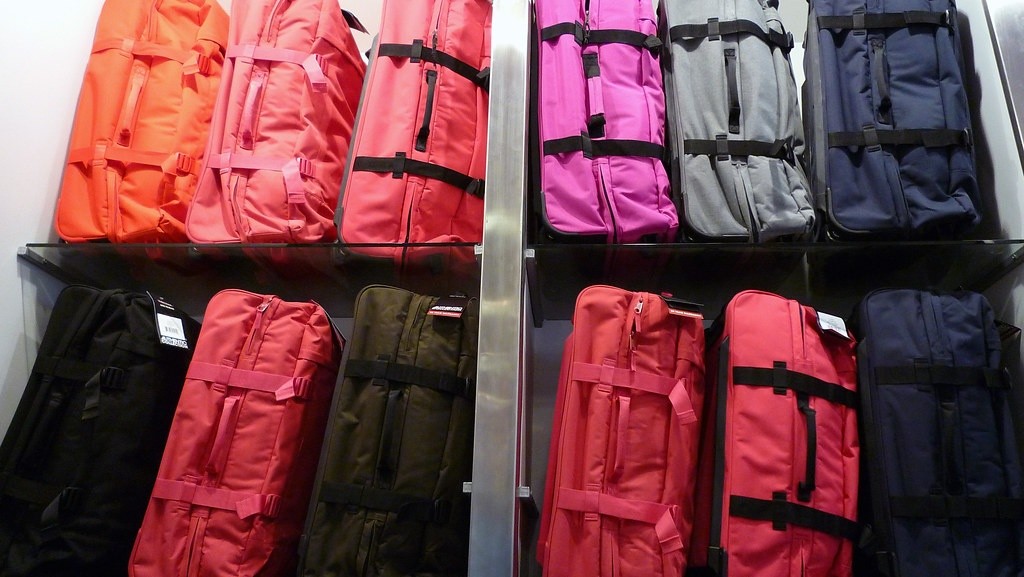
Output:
(0, 0), (1024, 577)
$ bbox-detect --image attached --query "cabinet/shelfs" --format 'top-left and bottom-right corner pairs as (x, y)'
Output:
(0, 0), (1024, 577)
